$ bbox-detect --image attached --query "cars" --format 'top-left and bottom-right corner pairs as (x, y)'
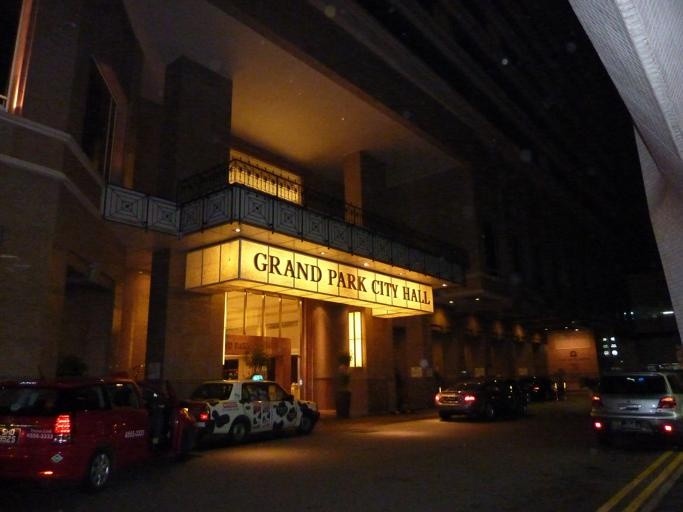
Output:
(431, 371), (570, 420)
(3, 377), (156, 490)
(135, 379), (215, 460)
(190, 379), (313, 447)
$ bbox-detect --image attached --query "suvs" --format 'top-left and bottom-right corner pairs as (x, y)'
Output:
(588, 369), (682, 446)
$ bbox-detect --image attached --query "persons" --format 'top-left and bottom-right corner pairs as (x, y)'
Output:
(333, 355), (354, 421)
